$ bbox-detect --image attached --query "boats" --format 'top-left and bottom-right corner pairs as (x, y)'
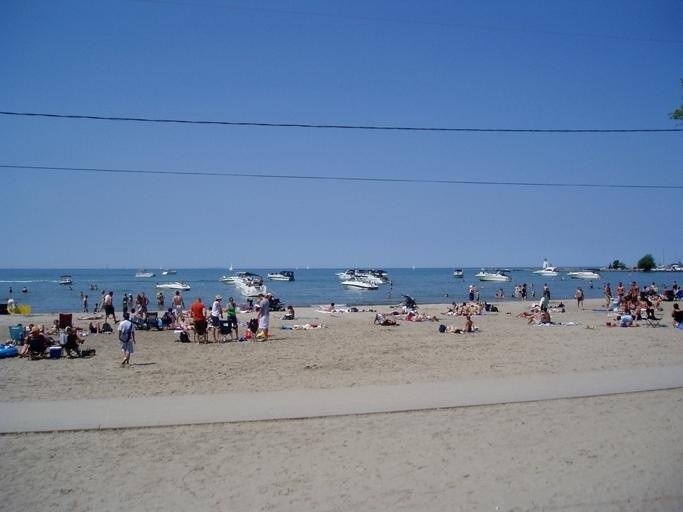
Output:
(267, 271), (294, 282)
(57, 275), (72, 285)
(234, 273), (266, 298)
(452, 268), (464, 278)
(335, 266), (391, 289)
(160, 269), (176, 275)
(474, 270), (511, 282)
(531, 258), (562, 277)
(22, 287), (28, 293)
(219, 271), (262, 286)
(565, 270), (600, 281)
(134, 271), (156, 278)
(495, 268), (510, 272)
(155, 280), (192, 291)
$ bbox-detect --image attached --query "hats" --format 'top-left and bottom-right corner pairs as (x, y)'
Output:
(216, 296), (222, 299)
(30, 326), (39, 334)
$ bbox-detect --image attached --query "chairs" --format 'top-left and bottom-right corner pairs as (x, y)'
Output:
(622, 287), (683, 329)
(5, 310), (235, 361)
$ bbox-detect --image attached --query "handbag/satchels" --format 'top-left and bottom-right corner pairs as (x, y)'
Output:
(119, 330), (131, 342)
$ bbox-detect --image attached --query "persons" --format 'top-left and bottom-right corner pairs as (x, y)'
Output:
(589, 280), (593, 288)
(373, 283), (566, 332)
(8, 282), (275, 365)
(318, 302), (335, 312)
(287, 305), (294, 318)
(604, 281), (683, 326)
(576, 287), (584, 310)
